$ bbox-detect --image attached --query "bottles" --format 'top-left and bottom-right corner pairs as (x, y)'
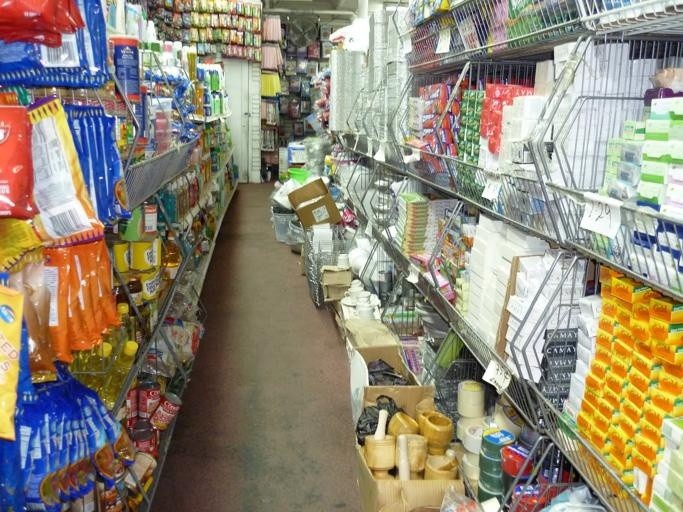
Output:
(65, 301), (141, 410)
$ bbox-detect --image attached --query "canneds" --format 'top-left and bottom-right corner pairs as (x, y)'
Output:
(106, 33), (148, 168)
(113, 205), (164, 343)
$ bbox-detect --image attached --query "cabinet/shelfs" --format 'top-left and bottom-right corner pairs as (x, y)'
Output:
(0, 0), (241, 511)
(331, 0), (683, 512)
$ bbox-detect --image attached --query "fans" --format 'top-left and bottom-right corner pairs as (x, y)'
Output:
(289, 16), (319, 48)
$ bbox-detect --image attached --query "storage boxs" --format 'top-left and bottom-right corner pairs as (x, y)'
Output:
(286, 177), (344, 231)
